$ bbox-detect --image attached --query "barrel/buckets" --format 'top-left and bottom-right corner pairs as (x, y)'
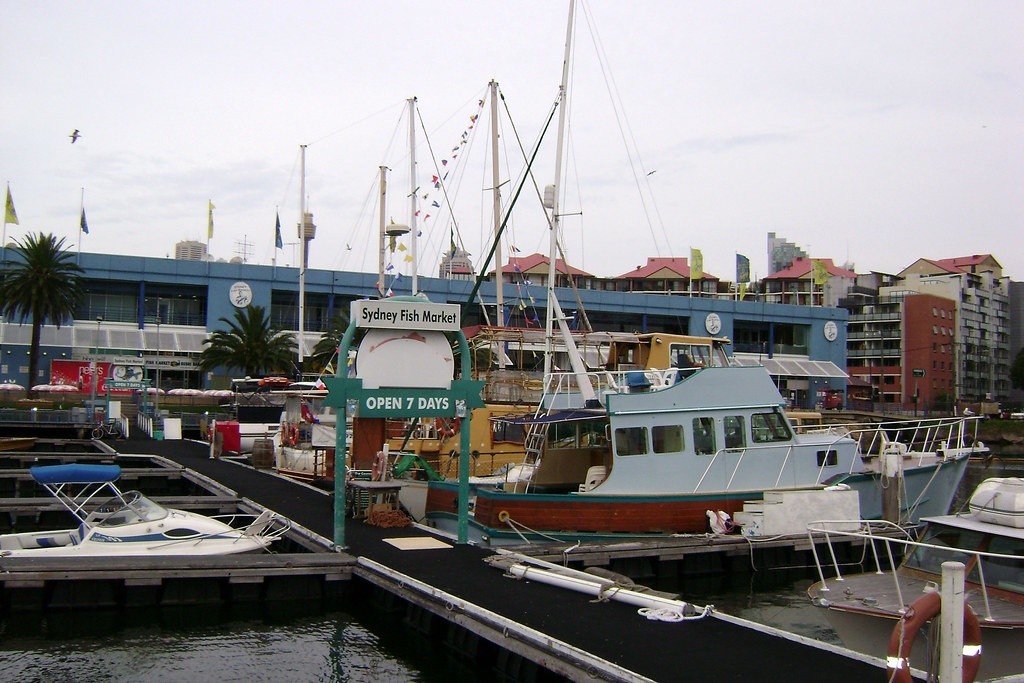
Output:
(252, 439), (274, 470)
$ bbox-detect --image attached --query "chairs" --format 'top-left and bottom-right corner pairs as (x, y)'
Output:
(579, 466), (606, 492)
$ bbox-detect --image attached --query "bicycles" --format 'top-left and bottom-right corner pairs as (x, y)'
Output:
(91, 418), (123, 441)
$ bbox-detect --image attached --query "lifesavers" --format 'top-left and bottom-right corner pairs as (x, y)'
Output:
(884, 592), (985, 683)
(436, 417), (460, 436)
(289, 424), (300, 446)
(281, 421), (289, 445)
(371, 450), (385, 480)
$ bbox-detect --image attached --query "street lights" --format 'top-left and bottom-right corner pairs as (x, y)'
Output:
(90, 315), (102, 412)
(155, 316), (162, 424)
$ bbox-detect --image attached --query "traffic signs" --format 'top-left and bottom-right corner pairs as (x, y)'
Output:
(913, 368), (925, 378)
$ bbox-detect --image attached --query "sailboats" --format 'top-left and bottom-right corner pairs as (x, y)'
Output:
(207, 0), (877, 522)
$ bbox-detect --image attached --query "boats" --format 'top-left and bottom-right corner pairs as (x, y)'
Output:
(806, 476), (1023, 681)
(428, 364), (989, 560)
(0, 463), (292, 556)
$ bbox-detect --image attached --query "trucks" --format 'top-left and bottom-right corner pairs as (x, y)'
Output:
(958, 401), (1004, 420)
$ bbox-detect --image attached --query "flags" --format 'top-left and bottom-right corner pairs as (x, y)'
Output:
(5, 188), (20, 226)
(81, 208), (90, 234)
(276, 214), (283, 248)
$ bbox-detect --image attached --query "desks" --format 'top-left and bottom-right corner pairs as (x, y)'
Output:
(349, 480), (408, 523)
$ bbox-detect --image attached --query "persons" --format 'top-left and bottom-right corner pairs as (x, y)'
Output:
(78, 372), (84, 390)
(755, 279), (766, 301)
(200, 415), (213, 441)
